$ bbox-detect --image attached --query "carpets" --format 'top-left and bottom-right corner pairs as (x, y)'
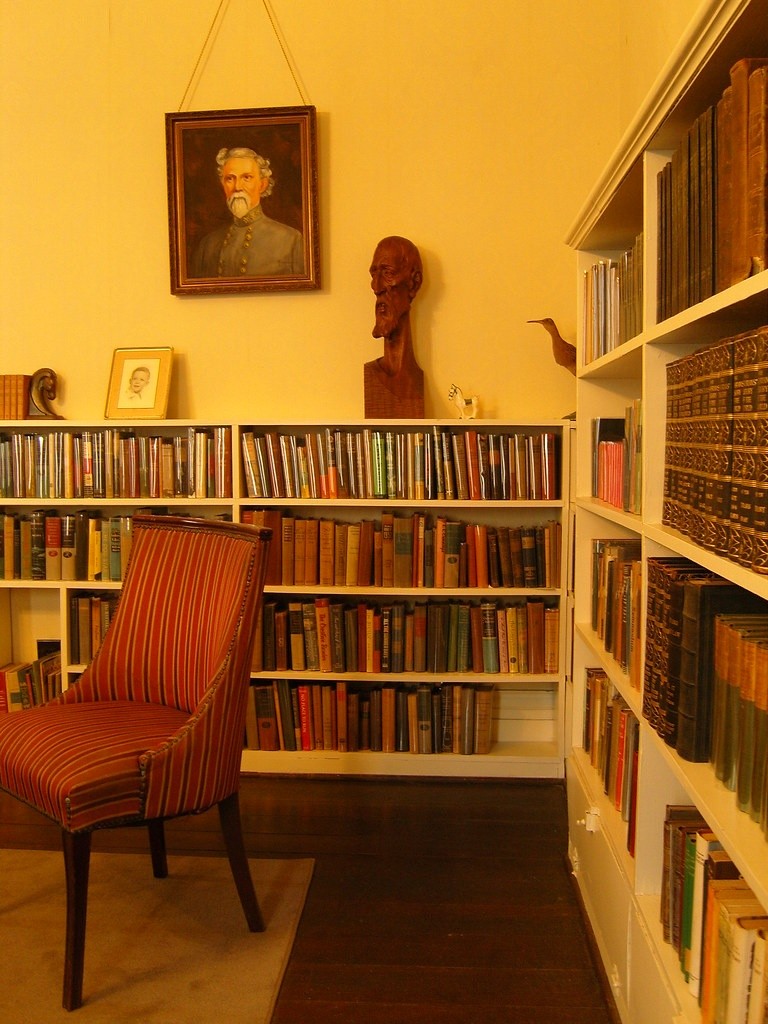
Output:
(1, 844), (317, 1023)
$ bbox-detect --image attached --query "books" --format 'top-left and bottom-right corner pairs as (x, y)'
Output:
(643, 558), (768, 843)
(241, 687), (500, 755)
(0, 428), (558, 505)
(68, 594), (559, 678)
(588, 536), (641, 694)
(584, 231), (654, 360)
(638, 667), (640, 857)
(585, 399), (641, 515)
(6, 638), (64, 713)
(665, 331), (768, 578)
(658, 801), (768, 1024)
(0, 511), (565, 594)
(652, 57), (768, 327)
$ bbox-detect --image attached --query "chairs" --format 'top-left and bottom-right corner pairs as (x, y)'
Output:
(1, 512), (275, 1013)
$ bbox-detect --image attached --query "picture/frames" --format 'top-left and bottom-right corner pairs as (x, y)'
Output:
(163, 105), (321, 296)
(102, 346), (174, 421)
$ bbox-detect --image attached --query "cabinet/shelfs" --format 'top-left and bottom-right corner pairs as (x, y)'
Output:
(560, 0), (767, 1023)
(1, 421), (565, 779)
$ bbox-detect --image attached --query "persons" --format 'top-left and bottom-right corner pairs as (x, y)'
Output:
(364, 236), (425, 419)
(115, 367), (154, 407)
